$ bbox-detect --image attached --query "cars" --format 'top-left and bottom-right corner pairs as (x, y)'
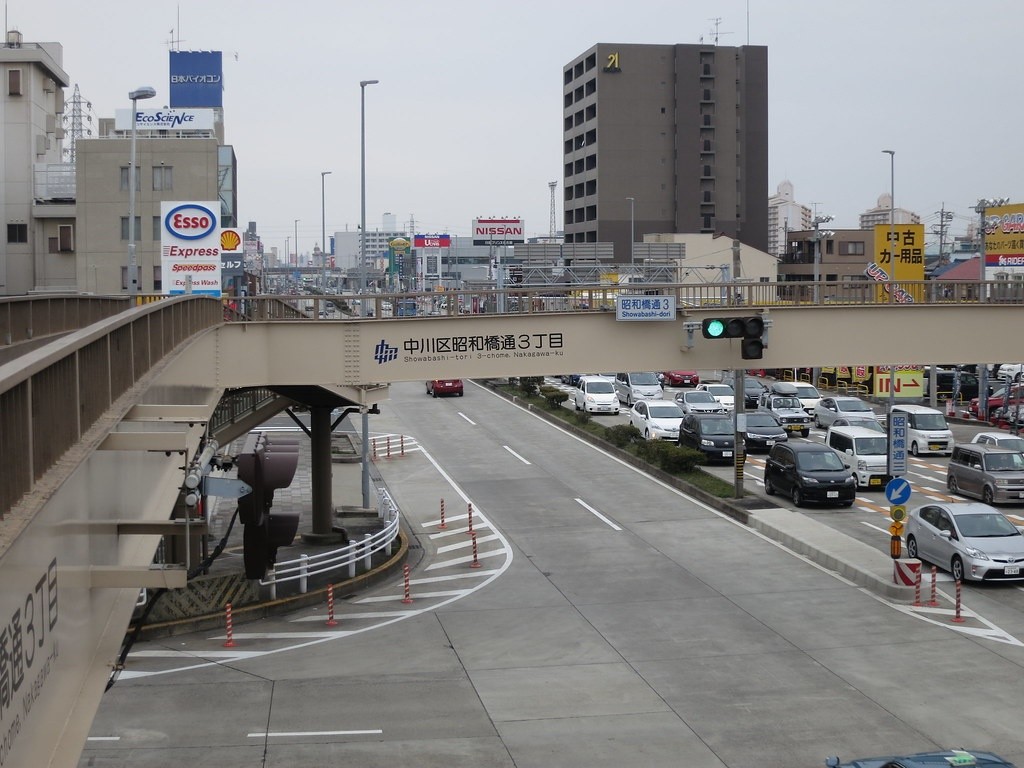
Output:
(276, 275), (487, 320)
(904, 502), (1024, 586)
(551, 364), (1024, 506)
(426, 379), (464, 398)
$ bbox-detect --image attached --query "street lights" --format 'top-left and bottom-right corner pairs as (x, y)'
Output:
(626, 197), (635, 294)
(974, 198), (1010, 302)
(778, 217), (795, 255)
(882, 149), (895, 303)
(287, 236), (292, 281)
(127, 86), (157, 310)
(809, 201), (836, 306)
(453, 234), (459, 290)
(359, 79), (379, 510)
(285, 240), (288, 275)
(322, 171), (332, 320)
(294, 219), (301, 303)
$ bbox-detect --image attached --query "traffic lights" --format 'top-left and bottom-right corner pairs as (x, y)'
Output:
(236, 432), (302, 502)
(740, 338), (764, 360)
(237, 492), (300, 579)
(702, 316), (764, 339)
